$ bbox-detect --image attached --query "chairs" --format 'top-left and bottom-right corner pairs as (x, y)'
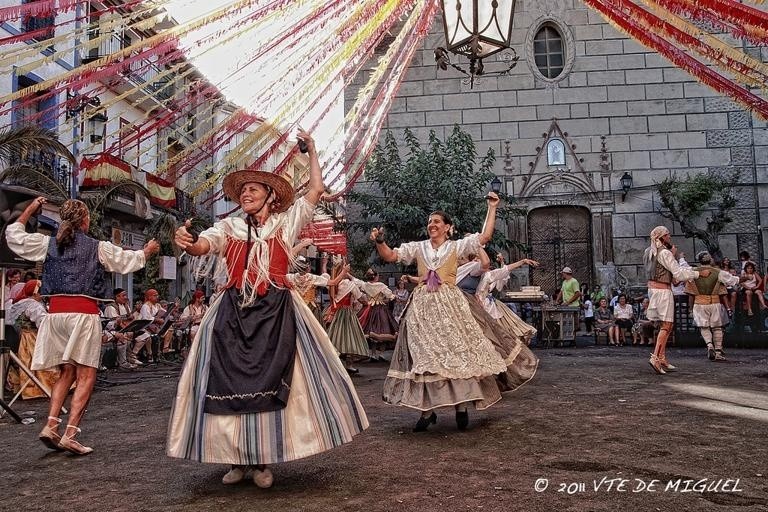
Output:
(593, 301), (675, 347)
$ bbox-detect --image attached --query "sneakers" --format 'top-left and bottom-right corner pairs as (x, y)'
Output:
(98, 366), (107, 372)
(223, 468), (249, 484)
(119, 354), (154, 369)
(708, 345), (725, 361)
(163, 348), (175, 353)
(609, 342), (653, 347)
(346, 367), (359, 373)
(727, 305), (767, 318)
(253, 468), (273, 488)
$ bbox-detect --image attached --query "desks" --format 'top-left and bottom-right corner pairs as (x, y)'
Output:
(500, 297), (544, 323)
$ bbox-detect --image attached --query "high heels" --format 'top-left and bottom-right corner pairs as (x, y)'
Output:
(455, 407), (468, 429)
(413, 412), (437, 432)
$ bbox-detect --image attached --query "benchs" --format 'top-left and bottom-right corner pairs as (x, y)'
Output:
(101, 334), (162, 368)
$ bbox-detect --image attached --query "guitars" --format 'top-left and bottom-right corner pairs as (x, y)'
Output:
(148, 295), (182, 334)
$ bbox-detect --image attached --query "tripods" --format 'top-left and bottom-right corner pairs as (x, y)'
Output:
(0, 269), (67, 418)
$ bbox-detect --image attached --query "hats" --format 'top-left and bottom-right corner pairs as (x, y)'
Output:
(560, 267), (572, 273)
(222, 169), (294, 213)
(113, 288), (125, 297)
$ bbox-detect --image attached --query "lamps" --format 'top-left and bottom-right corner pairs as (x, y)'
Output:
(433, 0), (520, 89)
(66, 90), (108, 143)
(619, 171), (632, 202)
(491, 177), (506, 200)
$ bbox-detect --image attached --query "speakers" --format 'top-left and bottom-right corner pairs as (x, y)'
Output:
(0, 183), (42, 269)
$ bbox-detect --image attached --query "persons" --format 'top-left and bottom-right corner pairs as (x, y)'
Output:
(456, 234), (537, 395)
(5, 196), (160, 455)
(0, 256), (408, 402)
(368, 189), (504, 432)
(163, 124), (369, 488)
(556, 251), (768, 347)
(682, 251), (752, 361)
(643, 226), (712, 374)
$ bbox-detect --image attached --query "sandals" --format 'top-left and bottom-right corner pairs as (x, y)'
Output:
(649, 355), (675, 374)
(39, 416), (63, 449)
(57, 425), (94, 455)
